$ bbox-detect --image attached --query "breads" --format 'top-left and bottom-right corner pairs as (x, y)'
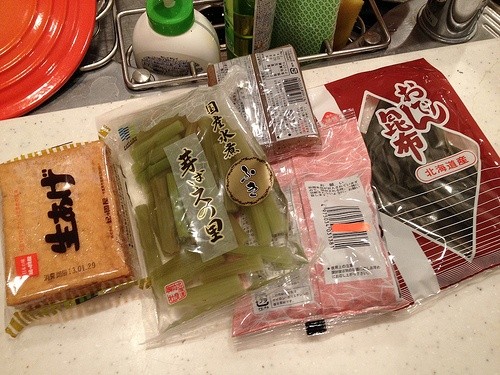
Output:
(0, 140), (138, 312)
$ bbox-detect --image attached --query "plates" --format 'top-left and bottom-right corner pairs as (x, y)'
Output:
(0, 0), (97, 121)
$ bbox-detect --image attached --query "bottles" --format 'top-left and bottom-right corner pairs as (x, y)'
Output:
(224, 0), (277, 58)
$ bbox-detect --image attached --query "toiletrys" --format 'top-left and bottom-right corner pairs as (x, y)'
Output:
(132, 0), (278, 78)
